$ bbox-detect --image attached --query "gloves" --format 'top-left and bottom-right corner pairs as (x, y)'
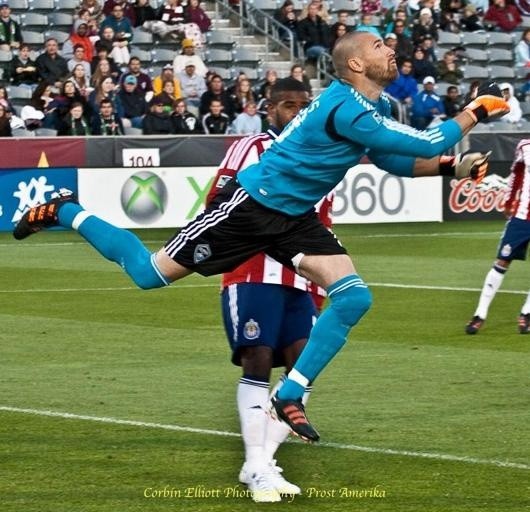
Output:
(463, 94), (510, 123)
(437, 149), (492, 183)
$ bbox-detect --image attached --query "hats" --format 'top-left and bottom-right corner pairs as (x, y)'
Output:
(124, 74), (135, 85)
(385, 32), (434, 53)
(423, 75), (435, 86)
(161, 39), (195, 69)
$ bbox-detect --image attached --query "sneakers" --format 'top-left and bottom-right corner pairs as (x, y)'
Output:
(264, 390), (320, 442)
(465, 314), (486, 335)
(518, 311), (530, 334)
(12, 188), (77, 240)
(246, 467), (282, 503)
(238, 462), (300, 494)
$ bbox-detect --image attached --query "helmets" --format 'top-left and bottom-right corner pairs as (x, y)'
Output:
(420, 7), (433, 17)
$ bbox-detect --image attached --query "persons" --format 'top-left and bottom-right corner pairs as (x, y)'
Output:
(206, 75), (334, 501)
(13, 30), (511, 441)
(465, 138), (530, 333)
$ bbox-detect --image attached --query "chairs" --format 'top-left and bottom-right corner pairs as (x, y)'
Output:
(0, 1), (529, 138)
